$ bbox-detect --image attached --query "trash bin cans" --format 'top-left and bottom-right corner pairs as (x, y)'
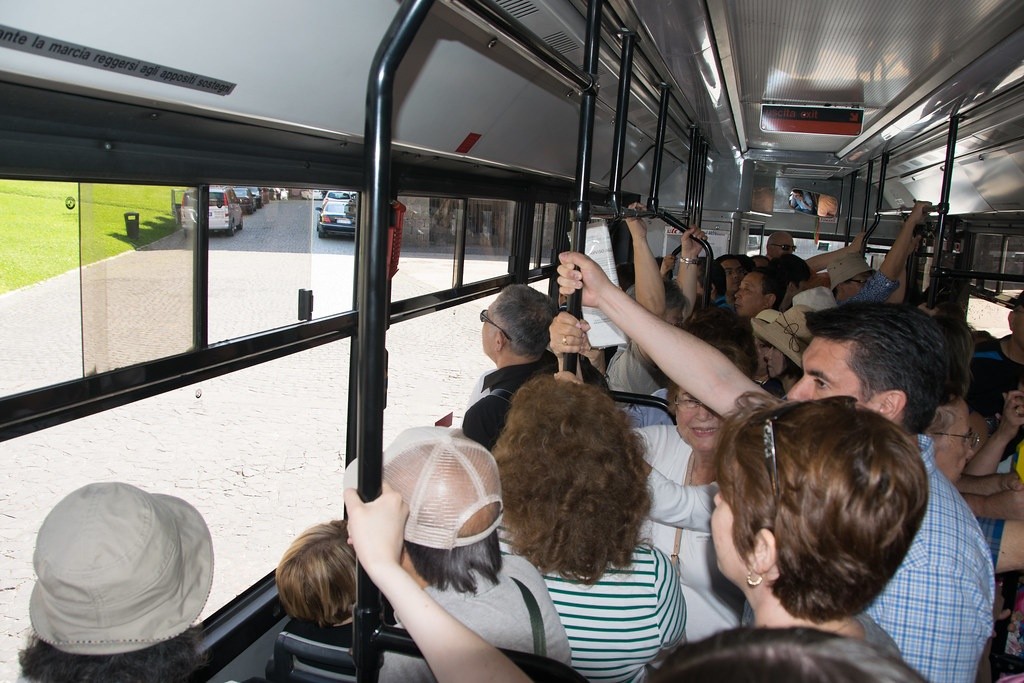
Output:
(124, 212), (139, 237)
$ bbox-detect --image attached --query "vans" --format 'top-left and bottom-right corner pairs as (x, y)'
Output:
(181, 185), (242, 236)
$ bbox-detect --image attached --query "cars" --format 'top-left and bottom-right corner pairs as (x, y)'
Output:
(248, 187), (289, 209)
(300, 189), (324, 200)
(233, 187), (254, 214)
(316, 200), (355, 240)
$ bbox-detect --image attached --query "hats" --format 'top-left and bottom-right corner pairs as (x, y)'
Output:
(29, 481), (217, 658)
(826, 250), (879, 290)
(751, 284), (840, 374)
(341, 423), (507, 548)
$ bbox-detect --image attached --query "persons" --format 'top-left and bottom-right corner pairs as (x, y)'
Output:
(16, 482), (214, 683)
(788, 190), (814, 213)
(275, 201), (1024, 683)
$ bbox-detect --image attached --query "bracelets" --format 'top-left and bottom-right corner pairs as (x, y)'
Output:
(680, 257), (697, 264)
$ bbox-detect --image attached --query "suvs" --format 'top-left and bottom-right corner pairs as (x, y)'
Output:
(321, 190), (359, 207)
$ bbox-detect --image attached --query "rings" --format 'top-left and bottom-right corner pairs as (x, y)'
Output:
(562, 337), (568, 345)
(1015, 410), (1020, 416)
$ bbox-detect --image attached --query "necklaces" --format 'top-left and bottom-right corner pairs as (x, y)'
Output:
(688, 459), (715, 486)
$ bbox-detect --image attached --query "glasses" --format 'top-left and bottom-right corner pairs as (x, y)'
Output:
(673, 394), (704, 411)
(480, 309), (512, 342)
(763, 392), (858, 502)
(931, 431), (982, 446)
(724, 268), (744, 277)
(770, 243), (798, 251)
(848, 278), (869, 286)
